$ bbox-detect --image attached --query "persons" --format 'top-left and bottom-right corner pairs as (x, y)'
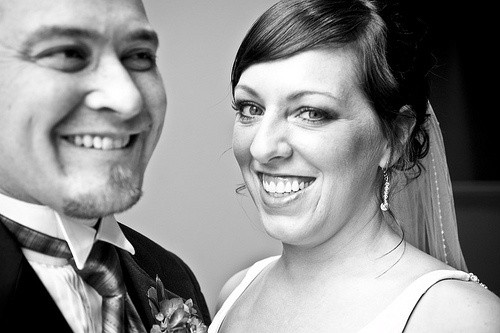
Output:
(1, 0), (212, 333)
(209, 0), (500, 333)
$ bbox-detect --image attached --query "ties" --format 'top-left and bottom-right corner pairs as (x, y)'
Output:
(2, 213), (159, 332)
